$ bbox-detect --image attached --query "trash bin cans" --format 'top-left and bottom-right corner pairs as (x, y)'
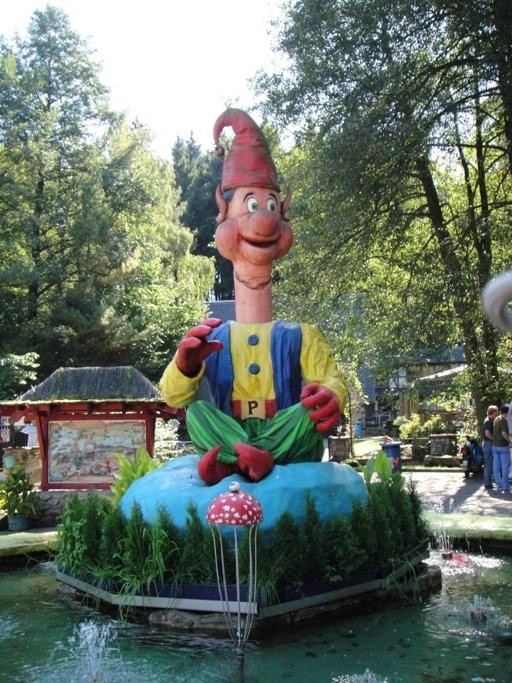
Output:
(14, 432), (29, 449)
(380, 442), (403, 475)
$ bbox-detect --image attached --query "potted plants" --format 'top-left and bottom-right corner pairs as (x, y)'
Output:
(0, 465), (36, 531)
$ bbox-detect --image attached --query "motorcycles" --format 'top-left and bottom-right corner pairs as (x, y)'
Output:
(461, 434), (484, 477)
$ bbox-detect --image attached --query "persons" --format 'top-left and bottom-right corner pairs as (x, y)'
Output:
(153, 106), (350, 487)
(20, 418), (37, 447)
(491, 405), (512, 493)
(481, 404), (500, 489)
